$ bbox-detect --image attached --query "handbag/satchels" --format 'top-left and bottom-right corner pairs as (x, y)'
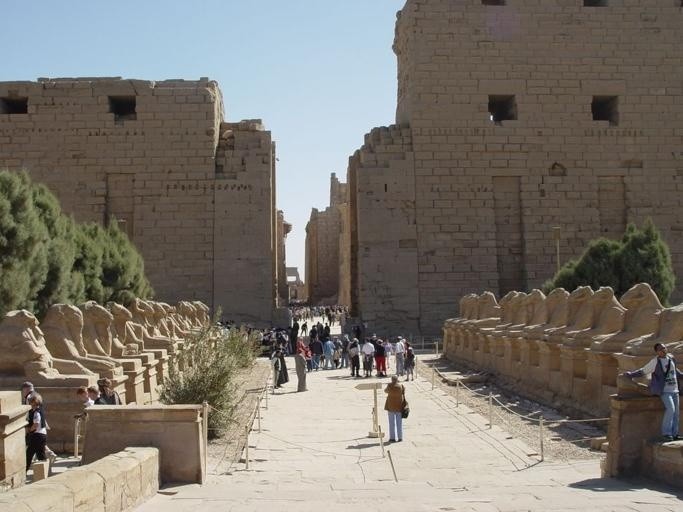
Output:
(401, 385), (410, 418)
(649, 358), (671, 396)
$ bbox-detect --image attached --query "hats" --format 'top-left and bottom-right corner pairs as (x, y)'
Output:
(376, 339), (384, 346)
(397, 336), (402, 339)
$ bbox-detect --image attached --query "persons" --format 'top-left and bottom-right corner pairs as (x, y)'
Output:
(72, 388), (94, 419)
(383, 375), (406, 443)
(22, 382), (34, 404)
(616, 342), (682, 441)
(86, 386), (107, 404)
(212, 301), (415, 391)
(25, 392), (46, 471)
(96, 377), (121, 404)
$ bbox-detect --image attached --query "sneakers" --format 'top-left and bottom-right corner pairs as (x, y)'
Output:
(662, 435), (673, 441)
(49, 454), (57, 464)
(674, 434), (683, 440)
